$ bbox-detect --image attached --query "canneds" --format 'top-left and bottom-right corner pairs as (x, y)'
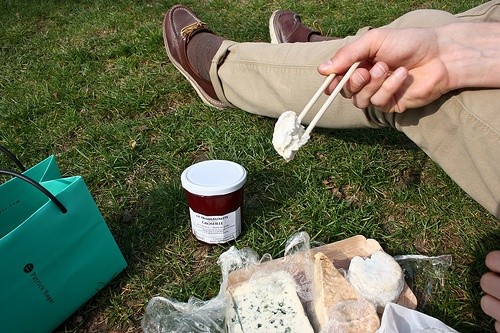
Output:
(181, 160), (247, 246)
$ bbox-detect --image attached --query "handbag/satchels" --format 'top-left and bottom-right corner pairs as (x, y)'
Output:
(0, 144), (129, 333)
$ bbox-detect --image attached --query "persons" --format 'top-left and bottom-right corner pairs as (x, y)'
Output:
(162, 0), (500, 333)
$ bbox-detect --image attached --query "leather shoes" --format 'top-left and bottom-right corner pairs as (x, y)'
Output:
(268, 8), (322, 44)
(162, 3), (229, 111)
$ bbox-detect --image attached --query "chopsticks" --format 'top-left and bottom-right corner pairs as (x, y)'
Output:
(298, 61), (360, 134)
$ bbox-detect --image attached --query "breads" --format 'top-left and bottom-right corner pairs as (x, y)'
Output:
(227, 250), (404, 333)
(272, 111), (310, 161)
